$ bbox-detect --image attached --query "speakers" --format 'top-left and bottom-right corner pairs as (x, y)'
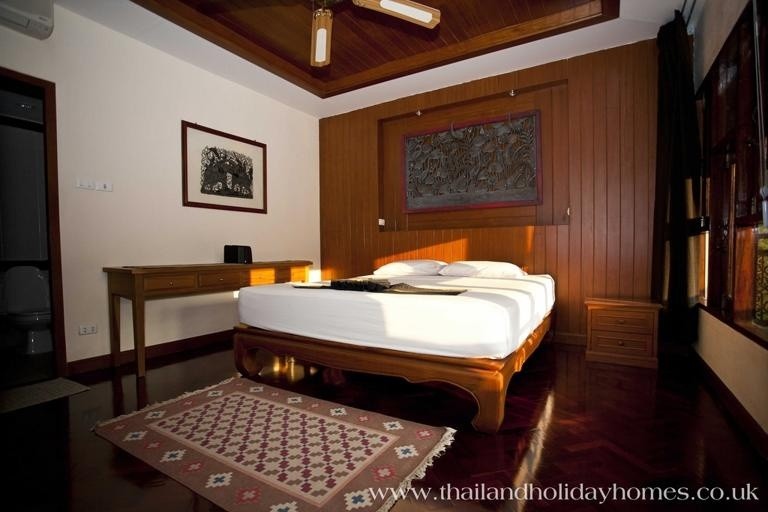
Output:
(224, 245), (252, 263)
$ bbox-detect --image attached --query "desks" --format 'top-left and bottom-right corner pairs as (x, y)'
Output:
(102, 260), (313, 376)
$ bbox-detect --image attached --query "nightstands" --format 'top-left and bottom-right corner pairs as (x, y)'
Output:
(584, 296), (664, 370)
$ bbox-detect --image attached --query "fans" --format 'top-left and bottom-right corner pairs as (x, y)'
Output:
(310, 1), (440, 68)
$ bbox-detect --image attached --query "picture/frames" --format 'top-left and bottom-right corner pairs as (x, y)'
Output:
(181, 121), (267, 214)
(400, 109), (543, 214)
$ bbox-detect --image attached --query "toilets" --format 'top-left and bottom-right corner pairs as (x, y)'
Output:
(0, 265), (54, 355)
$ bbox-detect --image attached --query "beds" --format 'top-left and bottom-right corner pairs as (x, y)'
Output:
(231, 275), (556, 432)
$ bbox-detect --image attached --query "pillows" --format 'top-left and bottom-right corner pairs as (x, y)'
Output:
(373, 260), (527, 279)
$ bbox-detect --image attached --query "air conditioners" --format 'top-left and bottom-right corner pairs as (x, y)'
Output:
(0, 1), (54, 40)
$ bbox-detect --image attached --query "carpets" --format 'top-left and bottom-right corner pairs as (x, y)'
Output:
(90, 372), (457, 511)
(0, 376), (91, 415)
